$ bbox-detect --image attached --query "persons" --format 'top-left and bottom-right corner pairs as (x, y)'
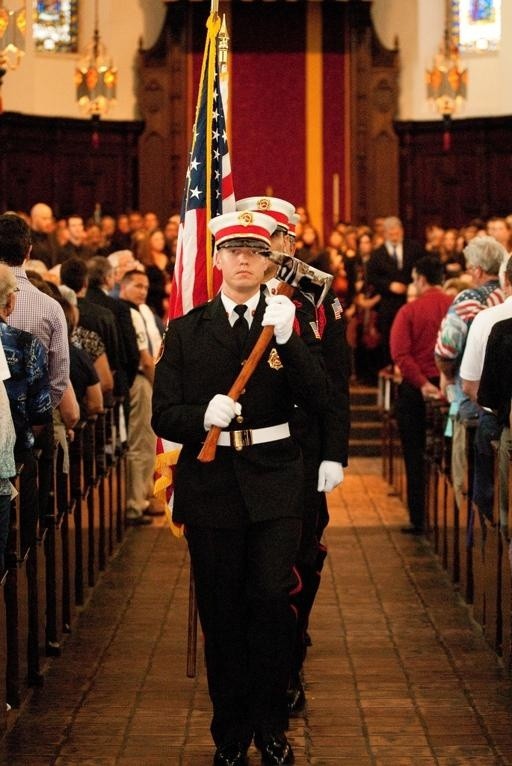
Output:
(151, 209), (351, 765)
(223, 192), (353, 723)
(0, 202), (179, 526)
(295, 207), (512, 536)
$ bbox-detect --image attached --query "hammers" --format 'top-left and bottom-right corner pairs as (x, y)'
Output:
(195, 249), (333, 463)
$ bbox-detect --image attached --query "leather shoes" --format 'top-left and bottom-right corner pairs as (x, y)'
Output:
(213, 739), (250, 766)
(284, 671), (306, 716)
(399, 524), (426, 537)
(142, 505), (167, 517)
(126, 515), (153, 526)
(253, 731), (298, 766)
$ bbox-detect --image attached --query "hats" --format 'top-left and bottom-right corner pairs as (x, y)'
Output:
(233, 195), (303, 236)
(207, 210), (279, 250)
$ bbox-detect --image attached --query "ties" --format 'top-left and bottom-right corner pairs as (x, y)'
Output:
(392, 245), (398, 265)
(231, 302), (250, 356)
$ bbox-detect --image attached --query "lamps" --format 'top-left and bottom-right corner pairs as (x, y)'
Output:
(0, 0), (26, 115)
(73, 0), (119, 148)
(423, 0), (469, 154)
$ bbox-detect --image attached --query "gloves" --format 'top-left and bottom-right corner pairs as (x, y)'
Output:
(316, 458), (344, 494)
(201, 393), (244, 430)
(258, 292), (299, 345)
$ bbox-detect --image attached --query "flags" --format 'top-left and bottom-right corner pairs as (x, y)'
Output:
(153, 7), (248, 540)
